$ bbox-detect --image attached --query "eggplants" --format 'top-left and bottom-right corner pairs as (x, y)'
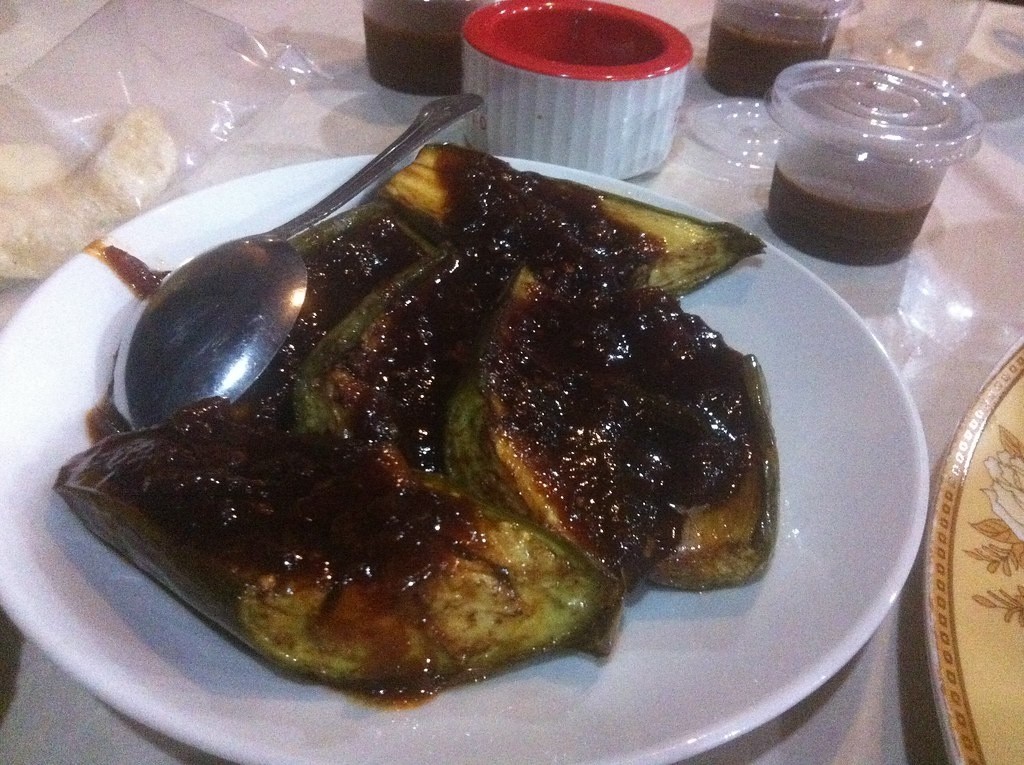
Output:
(52, 147), (781, 688)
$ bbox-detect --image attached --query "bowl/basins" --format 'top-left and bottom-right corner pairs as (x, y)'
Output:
(461, 0), (694, 180)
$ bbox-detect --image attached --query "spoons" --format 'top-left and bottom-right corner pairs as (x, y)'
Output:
(111, 90), (486, 436)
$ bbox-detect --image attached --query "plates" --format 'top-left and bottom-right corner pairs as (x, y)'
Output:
(925, 335), (1024, 765)
(0, 157), (930, 765)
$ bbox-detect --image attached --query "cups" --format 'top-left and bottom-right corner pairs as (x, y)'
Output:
(361, 0), (483, 97)
(853, 0), (985, 86)
(702, 0), (868, 102)
(760, 59), (986, 268)
